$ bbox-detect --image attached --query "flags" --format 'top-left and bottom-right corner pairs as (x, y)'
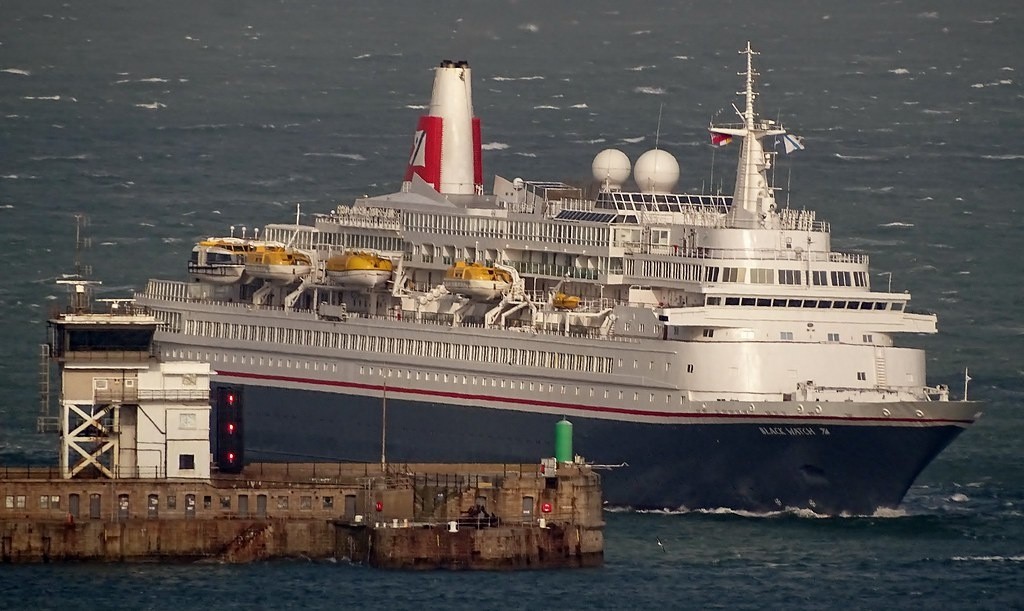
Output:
(710, 132), (733, 147)
(782, 134), (805, 154)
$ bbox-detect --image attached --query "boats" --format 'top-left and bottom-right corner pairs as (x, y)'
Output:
(44, 215), (166, 362)
(138, 36), (985, 520)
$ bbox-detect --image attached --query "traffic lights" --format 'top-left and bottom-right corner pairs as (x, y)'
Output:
(216, 385), (243, 475)
